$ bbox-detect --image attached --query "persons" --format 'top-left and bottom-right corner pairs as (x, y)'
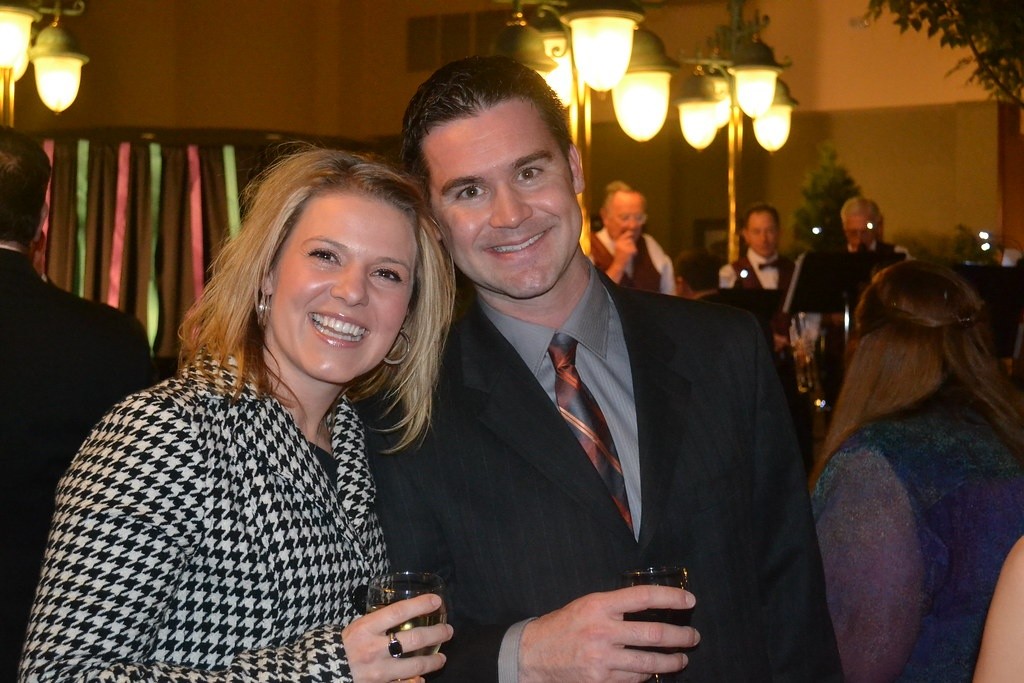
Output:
(355, 57), (846, 682)
(19, 147), (453, 683)
(0, 125), (154, 683)
(592, 181), (910, 462)
(811, 262), (1024, 682)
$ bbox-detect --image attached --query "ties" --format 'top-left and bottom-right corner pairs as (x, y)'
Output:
(547, 331), (635, 542)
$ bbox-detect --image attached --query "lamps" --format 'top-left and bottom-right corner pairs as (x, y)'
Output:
(675, 1), (800, 153)
(0, 0), (89, 116)
(506, 0), (680, 142)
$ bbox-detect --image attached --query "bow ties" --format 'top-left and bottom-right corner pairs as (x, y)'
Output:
(758, 261), (778, 271)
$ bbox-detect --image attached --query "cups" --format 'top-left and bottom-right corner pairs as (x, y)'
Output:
(622, 568), (692, 683)
(366, 573), (447, 683)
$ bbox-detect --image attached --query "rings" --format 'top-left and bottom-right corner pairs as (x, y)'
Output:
(388, 632), (402, 658)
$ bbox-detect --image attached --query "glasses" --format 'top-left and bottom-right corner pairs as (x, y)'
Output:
(612, 211), (647, 226)
